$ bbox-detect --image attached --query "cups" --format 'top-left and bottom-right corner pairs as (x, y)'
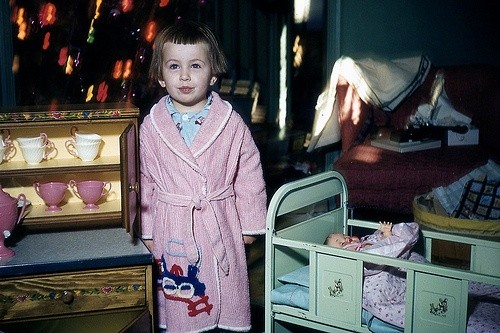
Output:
(65, 126), (102, 161)
(34, 182), (69, 212)
(17, 133), (55, 164)
(0, 129), (14, 164)
(70, 180), (111, 209)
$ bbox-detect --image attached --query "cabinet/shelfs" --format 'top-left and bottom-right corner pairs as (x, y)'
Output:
(0, 102), (142, 240)
(0, 225), (156, 333)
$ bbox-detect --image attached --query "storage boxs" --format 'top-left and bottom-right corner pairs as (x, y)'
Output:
(446, 126), (479, 146)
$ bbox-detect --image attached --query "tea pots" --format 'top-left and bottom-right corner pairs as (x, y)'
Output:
(0, 185), (26, 259)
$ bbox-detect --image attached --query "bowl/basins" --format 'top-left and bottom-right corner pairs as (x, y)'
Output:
(17, 199), (31, 212)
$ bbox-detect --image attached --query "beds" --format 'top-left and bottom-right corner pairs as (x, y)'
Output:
(264, 170), (500, 333)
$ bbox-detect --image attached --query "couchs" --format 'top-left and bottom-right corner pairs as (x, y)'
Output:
(331, 61), (500, 215)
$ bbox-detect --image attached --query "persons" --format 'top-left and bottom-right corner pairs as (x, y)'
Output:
(140, 21), (266, 333)
(323, 220), (393, 251)
